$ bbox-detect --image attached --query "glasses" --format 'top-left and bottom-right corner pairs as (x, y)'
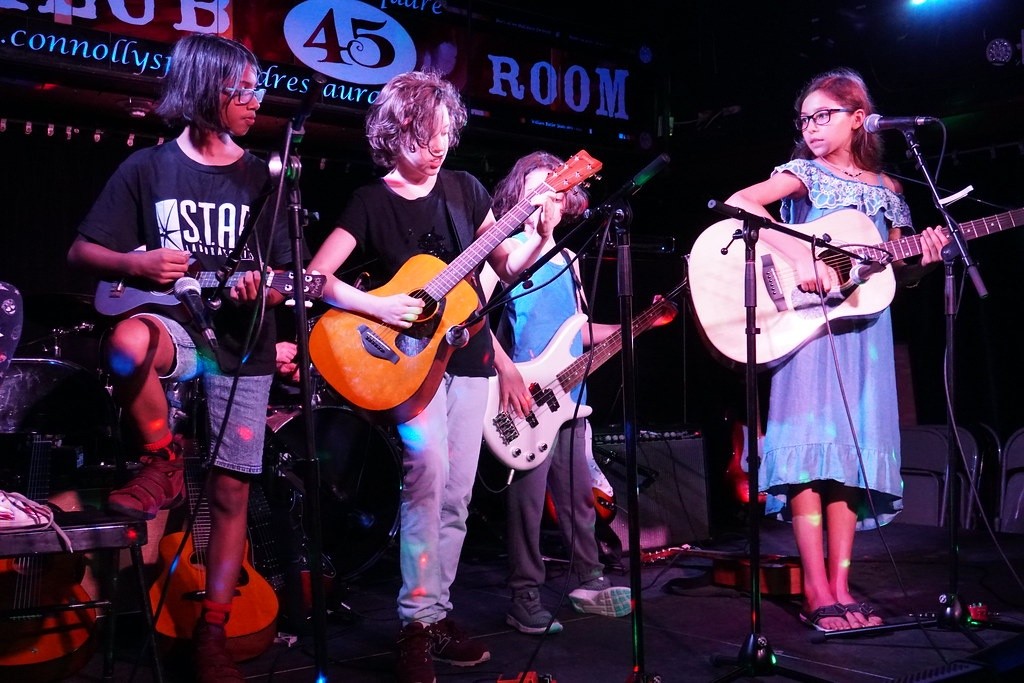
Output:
(219, 85), (267, 107)
(792, 108), (855, 130)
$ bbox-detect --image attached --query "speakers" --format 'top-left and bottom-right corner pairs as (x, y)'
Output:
(591, 429), (710, 554)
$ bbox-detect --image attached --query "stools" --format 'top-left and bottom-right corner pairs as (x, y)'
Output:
(0, 507), (168, 682)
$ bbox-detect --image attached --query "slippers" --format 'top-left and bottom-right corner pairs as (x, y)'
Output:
(799, 601), (850, 634)
(844, 599), (894, 637)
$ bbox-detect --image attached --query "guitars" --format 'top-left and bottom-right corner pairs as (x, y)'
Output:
(0, 429), (100, 673)
(138, 380), (281, 660)
(685, 204), (1024, 369)
(94, 246), (328, 320)
(479, 281), (687, 475)
(637, 544), (866, 605)
(307, 149), (605, 425)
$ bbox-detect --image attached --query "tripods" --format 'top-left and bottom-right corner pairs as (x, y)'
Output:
(709, 126), (1024, 683)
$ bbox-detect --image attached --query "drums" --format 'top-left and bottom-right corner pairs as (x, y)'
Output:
(267, 404), (408, 573)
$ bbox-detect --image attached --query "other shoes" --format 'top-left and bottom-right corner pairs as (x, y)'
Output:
(184, 600), (244, 683)
(111, 435), (188, 522)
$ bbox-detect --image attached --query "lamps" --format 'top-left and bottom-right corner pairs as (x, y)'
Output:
(985, 30), (1024, 65)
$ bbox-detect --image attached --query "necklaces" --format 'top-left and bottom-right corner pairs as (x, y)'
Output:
(821, 156), (863, 180)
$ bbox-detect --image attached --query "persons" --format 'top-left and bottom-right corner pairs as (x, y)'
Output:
(479, 151), (677, 633)
(67, 31), (312, 683)
(305, 72), (557, 683)
(724, 73), (949, 632)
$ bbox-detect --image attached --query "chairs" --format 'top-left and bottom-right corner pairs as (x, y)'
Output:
(893, 423), (1024, 534)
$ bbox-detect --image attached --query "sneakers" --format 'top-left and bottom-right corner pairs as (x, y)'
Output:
(393, 620), (436, 683)
(505, 589), (564, 634)
(430, 619), (491, 667)
(564, 576), (635, 619)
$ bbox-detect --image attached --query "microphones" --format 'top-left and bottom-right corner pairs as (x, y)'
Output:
(848, 253), (894, 284)
(446, 324), (470, 348)
(175, 276), (219, 352)
(863, 114), (940, 135)
(648, 471), (660, 481)
